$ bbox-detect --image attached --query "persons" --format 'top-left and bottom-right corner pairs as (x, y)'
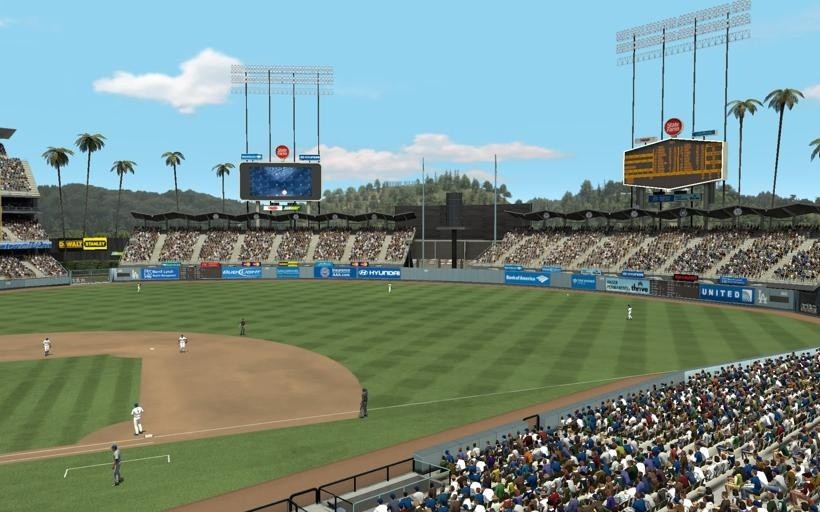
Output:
(122, 226), (413, 264)
(42, 338), (50, 354)
(112, 444), (121, 484)
(0, 155), (65, 282)
(178, 333), (186, 350)
(480, 221), (818, 289)
(358, 387), (369, 418)
(375, 349), (820, 512)
(628, 305), (633, 320)
(239, 318), (246, 335)
(131, 403), (145, 437)
(386, 282), (392, 292)
(137, 281), (140, 293)
